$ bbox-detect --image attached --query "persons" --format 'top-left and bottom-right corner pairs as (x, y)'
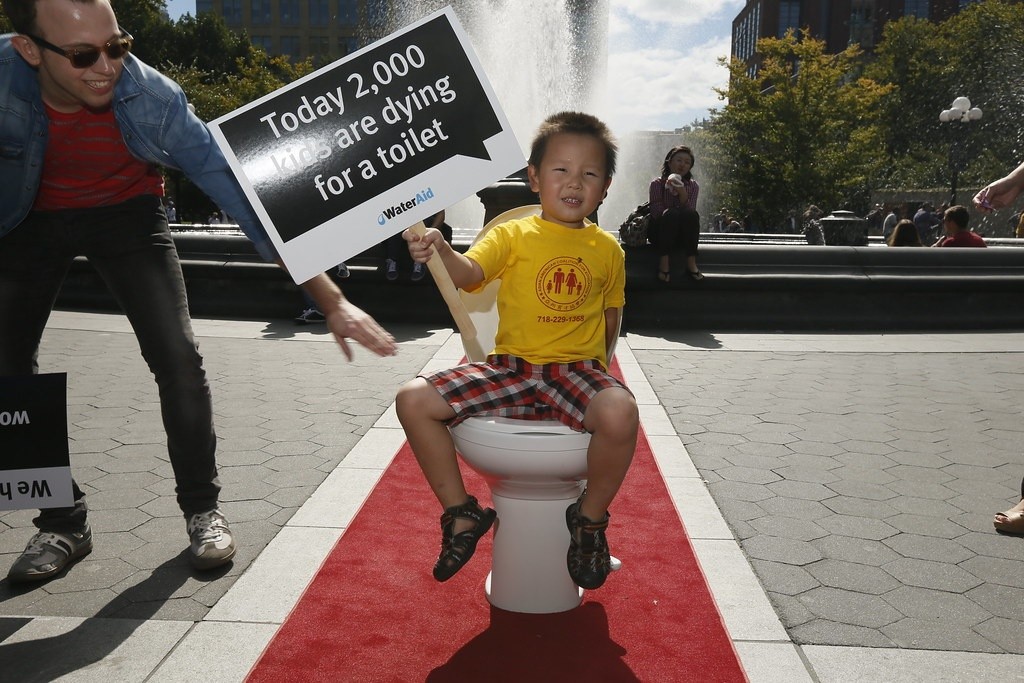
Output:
(649, 145), (704, 283)
(803, 205), (823, 226)
(714, 207), (742, 232)
(883, 207), (898, 241)
(0, 0), (398, 580)
(294, 260), (350, 322)
(395, 112), (639, 589)
(167, 201), (176, 224)
(941, 205), (987, 248)
(384, 210), (445, 281)
(888, 218), (922, 247)
(971, 162), (1024, 536)
(914, 202), (942, 245)
(208, 212), (220, 224)
(1007, 210), (1024, 238)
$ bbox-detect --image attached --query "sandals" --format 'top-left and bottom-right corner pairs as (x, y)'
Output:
(566, 489), (610, 589)
(993, 498), (1024, 534)
(433, 495), (496, 582)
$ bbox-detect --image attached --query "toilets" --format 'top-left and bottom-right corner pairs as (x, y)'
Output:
(449, 415), (600, 614)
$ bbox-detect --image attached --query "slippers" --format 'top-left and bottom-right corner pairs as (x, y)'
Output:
(656, 268), (671, 285)
(686, 266), (704, 281)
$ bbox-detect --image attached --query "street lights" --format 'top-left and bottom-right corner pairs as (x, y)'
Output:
(938, 97), (984, 206)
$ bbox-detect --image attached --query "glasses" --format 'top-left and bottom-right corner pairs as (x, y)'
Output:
(22, 24), (134, 68)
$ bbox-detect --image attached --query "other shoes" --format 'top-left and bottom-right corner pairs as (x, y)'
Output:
(335, 263), (350, 278)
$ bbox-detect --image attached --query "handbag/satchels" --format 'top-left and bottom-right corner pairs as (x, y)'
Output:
(620, 202), (651, 247)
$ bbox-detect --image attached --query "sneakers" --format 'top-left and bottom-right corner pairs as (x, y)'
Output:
(7, 522), (93, 584)
(185, 506), (237, 570)
(295, 308), (326, 323)
(410, 262), (426, 281)
(386, 257), (399, 280)
